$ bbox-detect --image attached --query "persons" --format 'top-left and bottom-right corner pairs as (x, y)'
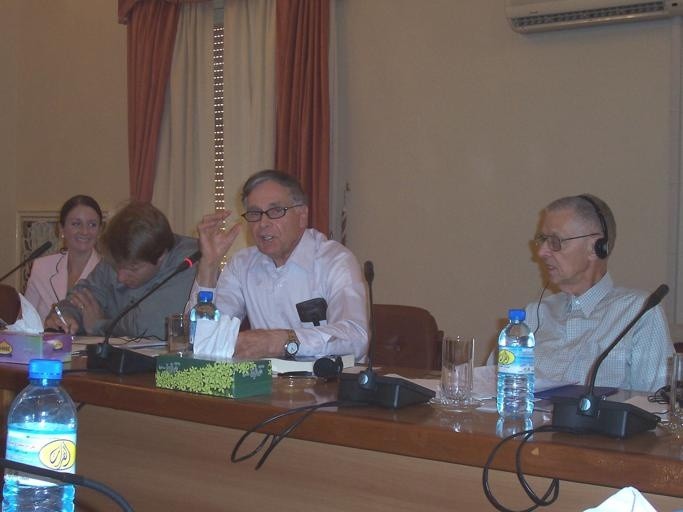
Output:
(24, 195), (108, 333)
(184, 169), (370, 364)
(44, 201), (202, 343)
(487, 194), (677, 393)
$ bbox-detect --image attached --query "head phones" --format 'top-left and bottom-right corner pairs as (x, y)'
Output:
(576, 195), (609, 261)
(314, 356), (342, 381)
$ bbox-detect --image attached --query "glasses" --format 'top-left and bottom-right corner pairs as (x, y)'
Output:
(241, 203), (304, 223)
(535, 232), (601, 251)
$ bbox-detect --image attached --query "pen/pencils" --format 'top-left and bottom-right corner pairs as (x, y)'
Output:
(55, 307), (73, 340)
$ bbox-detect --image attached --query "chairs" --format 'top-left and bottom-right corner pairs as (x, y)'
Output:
(366, 303), (443, 371)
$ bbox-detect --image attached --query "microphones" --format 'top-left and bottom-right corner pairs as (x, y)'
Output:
(103, 249), (204, 341)
(586, 283), (669, 400)
(364, 261), (374, 374)
(0, 240), (52, 283)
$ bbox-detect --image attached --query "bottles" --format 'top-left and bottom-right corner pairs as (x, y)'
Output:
(3, 359), (76, 512)
(187, 291), (220, 350)
(496, 416), (535, 441)
(496, 310), (534, 418)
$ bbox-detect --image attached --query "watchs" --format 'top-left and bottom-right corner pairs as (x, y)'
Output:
(284, 329), (298, 360)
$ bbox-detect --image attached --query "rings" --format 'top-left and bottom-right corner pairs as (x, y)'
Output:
(80, 305), (84, 310)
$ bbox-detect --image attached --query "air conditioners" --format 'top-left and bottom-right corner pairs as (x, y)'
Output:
(502, 0), (682, 35)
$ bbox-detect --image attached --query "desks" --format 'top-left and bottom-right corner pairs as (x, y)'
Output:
(0, 330), (683, 512)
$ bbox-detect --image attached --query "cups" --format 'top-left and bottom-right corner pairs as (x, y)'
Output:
(667, 352), (683, 441)
(167, 314), (191, 358)
(441, 336), (475, 408)
